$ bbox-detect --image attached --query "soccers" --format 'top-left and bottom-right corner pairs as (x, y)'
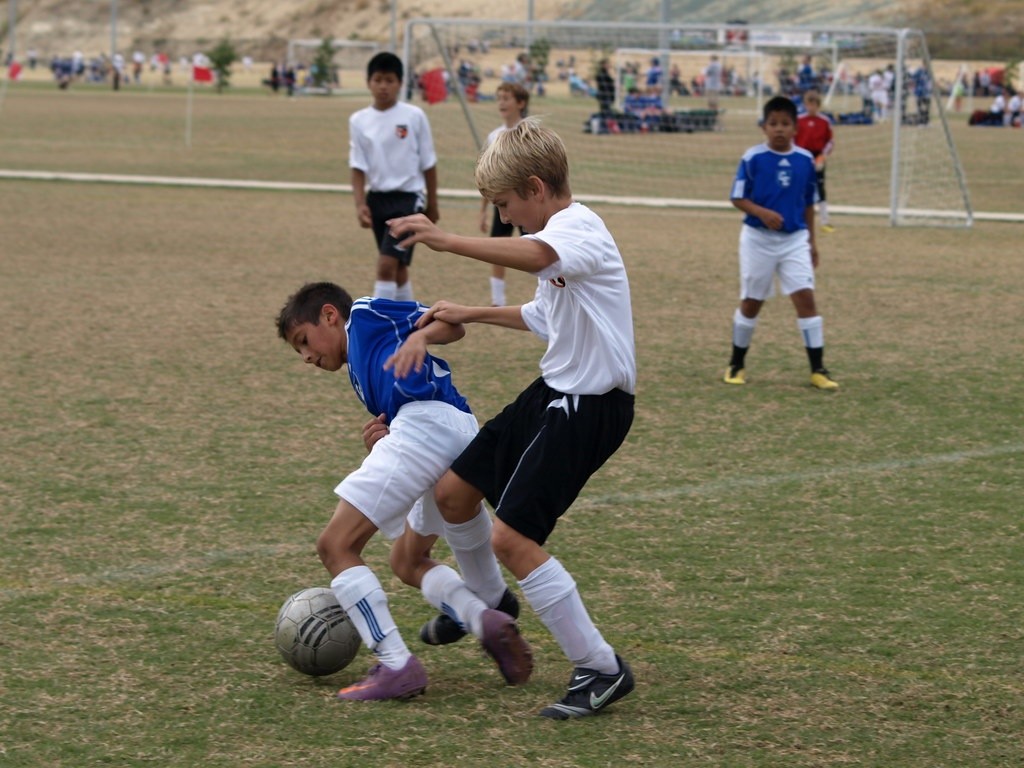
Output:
(275, 586), (361, 675)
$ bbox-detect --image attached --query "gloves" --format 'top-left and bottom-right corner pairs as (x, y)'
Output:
(816, 156), (825, 171)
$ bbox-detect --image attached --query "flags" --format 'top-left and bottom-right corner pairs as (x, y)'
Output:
(193, 65), (212, 82)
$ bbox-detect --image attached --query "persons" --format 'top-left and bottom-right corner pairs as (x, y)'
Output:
(348, 52), (440, 301)
(6, 44), (306, 98)
(479, 83), (529, 306)
(276, 121), (637, 721)
(725, 95), (840, 390)
(406, 55), (527, 101)
(556, 47), (936, 125)
(952, 66), (1022, 127)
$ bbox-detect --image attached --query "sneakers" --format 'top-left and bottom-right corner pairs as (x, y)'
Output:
(338, 654), (427, 700)
(810, 368), (840, 389)
(421, 586), (520, 645)
(541, 653), (635, 719)
(724, 365), (746, 384)
(479, 608), (534, 686)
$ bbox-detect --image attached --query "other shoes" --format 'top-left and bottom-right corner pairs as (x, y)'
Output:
(821, 224), (836, 233)
